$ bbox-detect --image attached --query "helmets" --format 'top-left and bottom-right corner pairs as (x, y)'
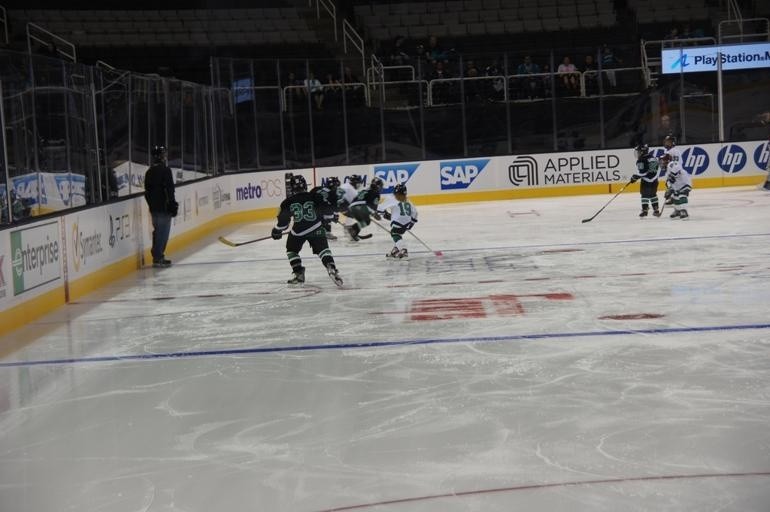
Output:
(636, 135), (677, 166)
(152, 145), (169, 156)
(371, 177), (382, 191)
(290, 175), (307, 192)
(394, 183), (407, 195)
(350, 175), (362, 185)
(326, 177), (340, 187)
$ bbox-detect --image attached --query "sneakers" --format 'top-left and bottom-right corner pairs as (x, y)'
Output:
(152, 255), (171, 264)
(347, 227), (359, 241)
(653, 211), (660, 216)
(287, 267), (306, 283)
(665, 198), (672, 204)
(670, 208), (689, 219)
(386, 246), (408, 258)
(326, 231), (337, 239)
(638, 211), (648, 217)
(326, 262), (343, 285)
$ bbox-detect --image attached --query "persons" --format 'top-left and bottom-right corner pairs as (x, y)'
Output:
(1, 187), (31, 228)
(85, 150), (120, 208)
(660, 136), (683, 204)
(659, 153), (693, 221)
(386, 34), (625, 102)
(657, 115), (682, 145)
(143, 145), (180, 267)
(286, 64), (364, 112)
(272, 173), (418, 289)
(630, 143), (660, 218)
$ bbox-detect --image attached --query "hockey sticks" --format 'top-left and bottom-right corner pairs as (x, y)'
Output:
(219, 232), (291, 247)
(338, 221), (372, 239)
(657, 198), (666, 217)
(582, 181), (632, 222)
(405, 228), (442, 255)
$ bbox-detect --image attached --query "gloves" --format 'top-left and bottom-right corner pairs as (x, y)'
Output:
(384, 210), (391, 220)
(271, 227), (283, 239)
(167, 201), (178, 216)
(406, 216), (418, 230)
